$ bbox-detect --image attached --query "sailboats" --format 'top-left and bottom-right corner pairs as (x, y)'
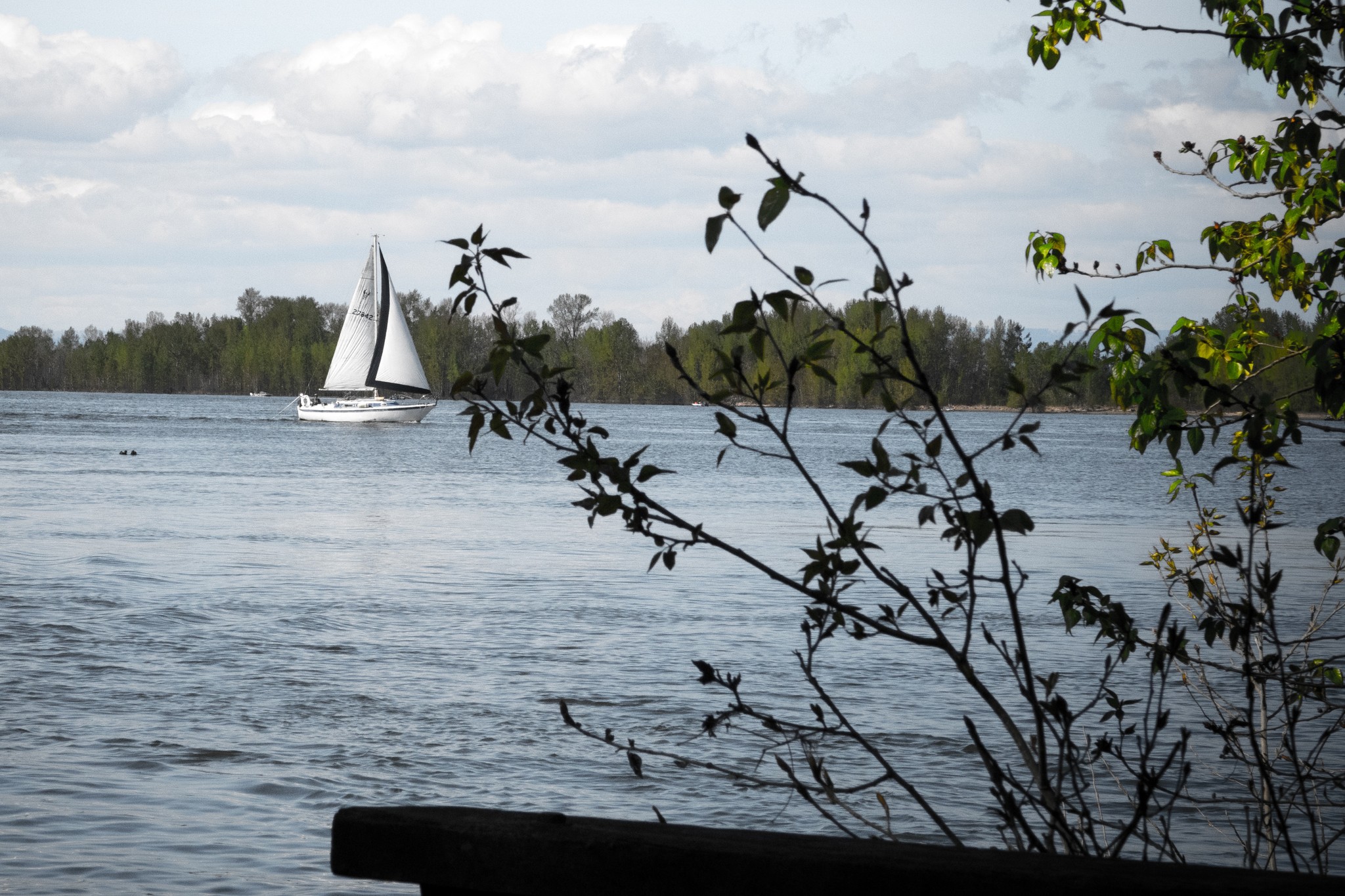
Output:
(297, 232), (440, 423)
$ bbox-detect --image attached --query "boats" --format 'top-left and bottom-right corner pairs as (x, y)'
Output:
(692, 402), (705, 407)
(249, 391), (271, 397)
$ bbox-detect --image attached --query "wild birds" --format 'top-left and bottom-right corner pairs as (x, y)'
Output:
(131, 450), (137, 455)
(120, 450), (127, 455)
